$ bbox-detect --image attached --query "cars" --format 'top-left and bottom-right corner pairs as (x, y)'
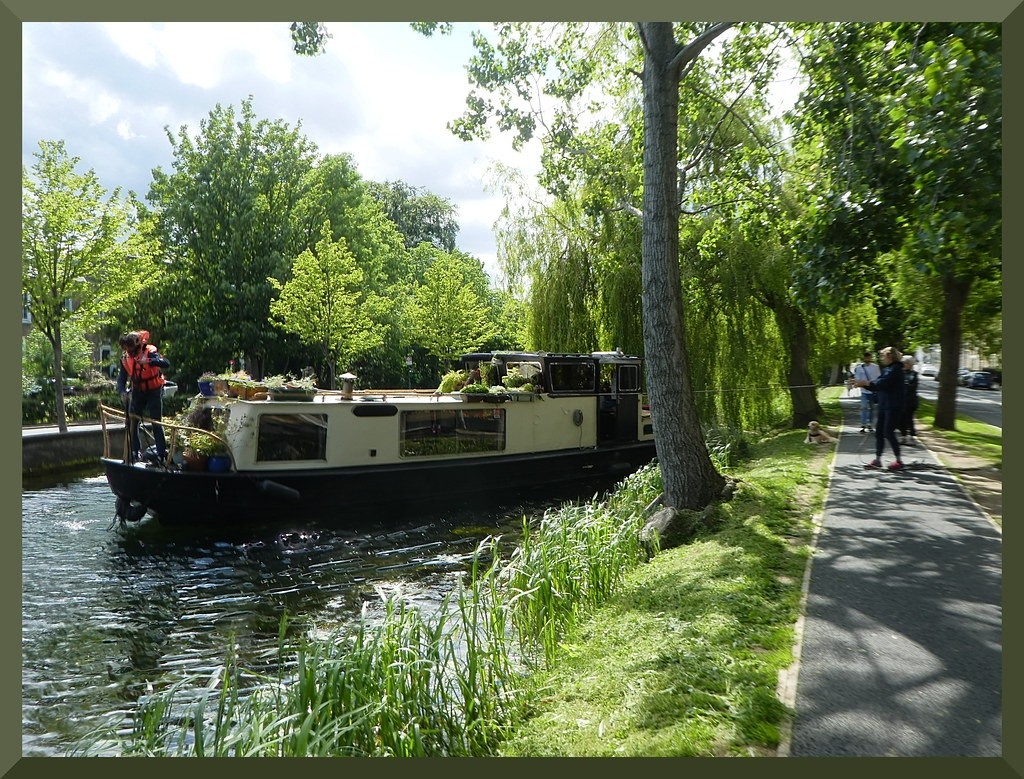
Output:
(957, 370), (993, 389)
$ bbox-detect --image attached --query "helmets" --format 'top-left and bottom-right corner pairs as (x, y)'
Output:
(119, 329), (141, 352)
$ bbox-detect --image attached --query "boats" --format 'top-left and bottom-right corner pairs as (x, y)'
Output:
(96, 347), (658, 536)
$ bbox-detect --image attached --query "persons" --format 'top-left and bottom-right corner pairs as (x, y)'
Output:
(854, 347), (919, 469)
(116, 329), (170, 467)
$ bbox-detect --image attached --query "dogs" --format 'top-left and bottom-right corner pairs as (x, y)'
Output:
(803, 421), (839, 444)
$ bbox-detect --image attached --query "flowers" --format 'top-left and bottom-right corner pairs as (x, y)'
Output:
(198, 360), (234, 381)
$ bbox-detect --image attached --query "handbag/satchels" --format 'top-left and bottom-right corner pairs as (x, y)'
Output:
(872, 391), (879, 403)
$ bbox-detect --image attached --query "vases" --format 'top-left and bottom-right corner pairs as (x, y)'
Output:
(214, 381), (227, 396)
(196, 381), (213, 396)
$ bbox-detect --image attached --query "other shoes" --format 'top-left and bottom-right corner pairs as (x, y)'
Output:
(864, 460), (882, 470)
(869, 426), (875, 434)
(888, 460), (905, 472)
(897, 434), (915, 446)
(860, 426), (865, 434)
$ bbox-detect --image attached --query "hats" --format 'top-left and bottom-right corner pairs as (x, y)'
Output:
(863, 351), (871, 355)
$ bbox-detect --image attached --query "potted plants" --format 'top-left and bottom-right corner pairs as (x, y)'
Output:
(228, 366), (318, 401)
(146, 395), (243, 471)
(435, 362), (539, 403)
